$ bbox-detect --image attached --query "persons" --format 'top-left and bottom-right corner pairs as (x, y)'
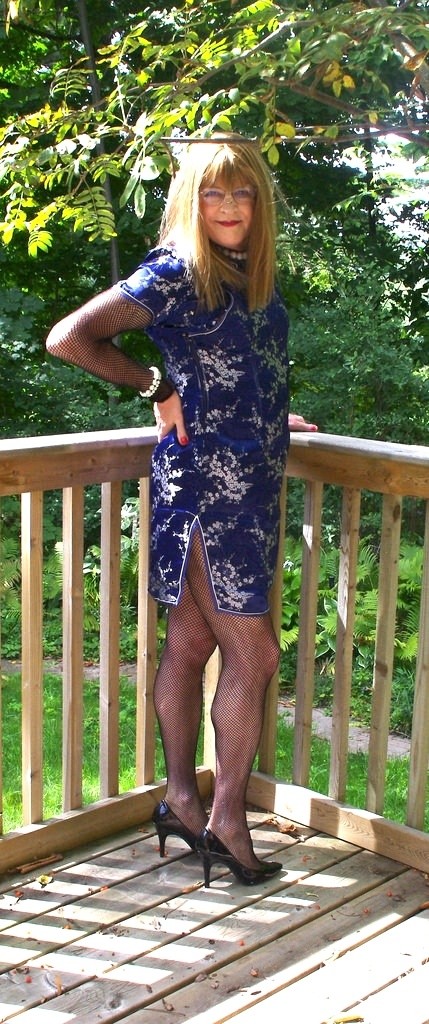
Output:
(46, 132), (318, 887)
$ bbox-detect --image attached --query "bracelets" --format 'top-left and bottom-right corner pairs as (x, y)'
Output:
(139, 366), (162, 398)
(150, 378), (175, 402)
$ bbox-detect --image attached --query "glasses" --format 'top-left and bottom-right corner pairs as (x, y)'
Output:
(200, 187), (257, 206)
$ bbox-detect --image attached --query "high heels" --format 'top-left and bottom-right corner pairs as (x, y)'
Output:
(152, 800), (206, 859)
(201, 829), (283, 888)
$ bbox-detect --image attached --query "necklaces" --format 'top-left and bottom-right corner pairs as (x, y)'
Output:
(216, 247), (247, 260)
